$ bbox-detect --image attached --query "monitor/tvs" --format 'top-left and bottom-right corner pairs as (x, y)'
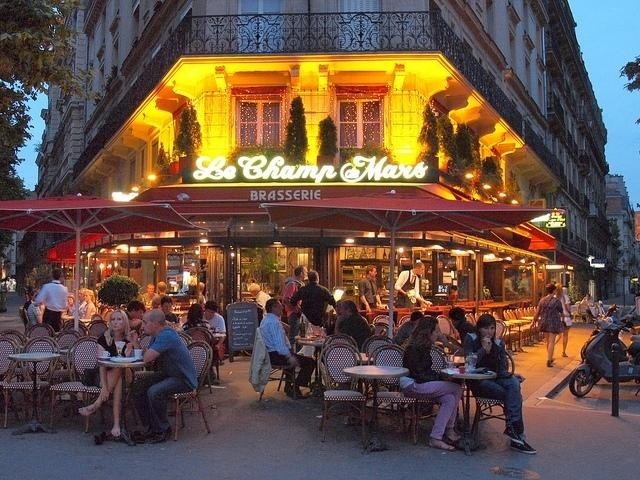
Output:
(437, 283), (450, 294)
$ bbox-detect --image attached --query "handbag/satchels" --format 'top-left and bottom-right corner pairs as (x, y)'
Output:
(538, 318), (547, 333)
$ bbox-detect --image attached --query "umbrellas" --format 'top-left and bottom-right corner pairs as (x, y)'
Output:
(0, 192), (212, 331)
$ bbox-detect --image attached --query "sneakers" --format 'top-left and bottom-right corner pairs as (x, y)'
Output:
(502, 426), (538, 455)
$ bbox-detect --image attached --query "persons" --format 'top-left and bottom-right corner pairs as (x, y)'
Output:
(247, 283), (272, 309)
(25, 269), (226, 444)
(261, 297), (316, 399)
(447, 286), (459, 300)
(290, 270), (336, 381)
(552, 283), (572, 357)
(504, 269), (531, 302)
(530, 283), (566, 368)
(282, 266), (306, 345)
(394, 262), (433, 306)
(333, 299), (371, 349)
(357, 265), (382, 314)
(394, 306), (538, 454)
(576, 294), (605, 323)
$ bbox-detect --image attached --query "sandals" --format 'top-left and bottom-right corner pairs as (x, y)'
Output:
(429, 439), (456, 451)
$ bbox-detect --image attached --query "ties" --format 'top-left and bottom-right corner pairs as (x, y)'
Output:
(369, 280), (377, 304)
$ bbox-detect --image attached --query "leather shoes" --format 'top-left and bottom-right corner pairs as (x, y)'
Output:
(132, 428), (171, 444)
(94, 430), (106, 445)
(284, 382), (307, 399)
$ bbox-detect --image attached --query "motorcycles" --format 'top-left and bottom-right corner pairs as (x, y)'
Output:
(569, 304), (640, 403)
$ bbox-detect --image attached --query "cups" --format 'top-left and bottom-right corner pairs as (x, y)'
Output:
(98, 340), (145, 363)
(444, 350), (479, 375)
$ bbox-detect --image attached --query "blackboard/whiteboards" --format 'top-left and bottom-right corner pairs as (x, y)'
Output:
(226, 302), (258, 351)
